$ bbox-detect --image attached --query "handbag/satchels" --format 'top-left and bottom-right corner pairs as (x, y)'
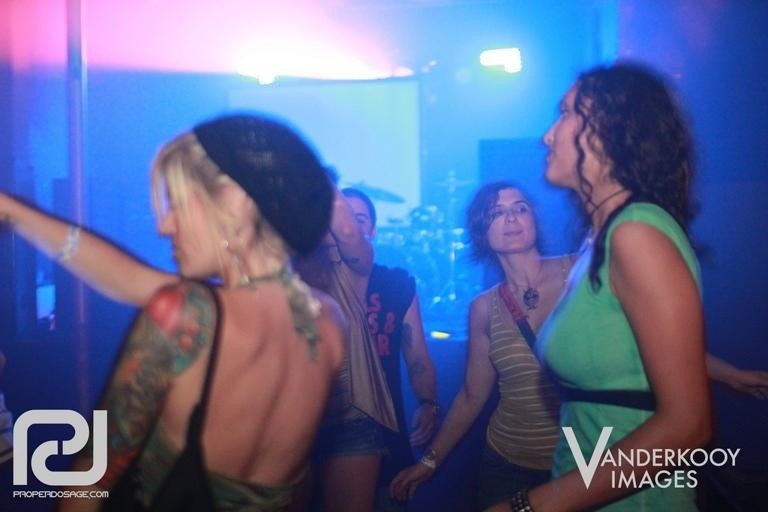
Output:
(101, 277), (222, 512)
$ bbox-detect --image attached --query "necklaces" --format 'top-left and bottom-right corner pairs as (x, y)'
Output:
(574, 184), (629, 258)
(500, 255), (547, 311)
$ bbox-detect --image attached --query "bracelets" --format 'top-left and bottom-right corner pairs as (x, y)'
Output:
(508, 485), (534, 511)
(49, 220), (81, 265)
(417, 444), (444, 474)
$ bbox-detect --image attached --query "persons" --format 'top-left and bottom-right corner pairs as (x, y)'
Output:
(483, 59), (716, 510)
(338, 188), (442, 511)
(292, 161), (403, 512)
(0, 111), (352, 509)
(385, 179), (767, 511)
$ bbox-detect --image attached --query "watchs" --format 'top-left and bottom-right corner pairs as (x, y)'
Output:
(419, 397), (441, 418)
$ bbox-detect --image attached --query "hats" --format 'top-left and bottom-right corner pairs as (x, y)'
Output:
(192, 114), (333, 259)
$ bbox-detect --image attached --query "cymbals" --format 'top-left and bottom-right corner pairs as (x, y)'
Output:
(430, 180), (467, 191)
(352, 184), (403, 204)
